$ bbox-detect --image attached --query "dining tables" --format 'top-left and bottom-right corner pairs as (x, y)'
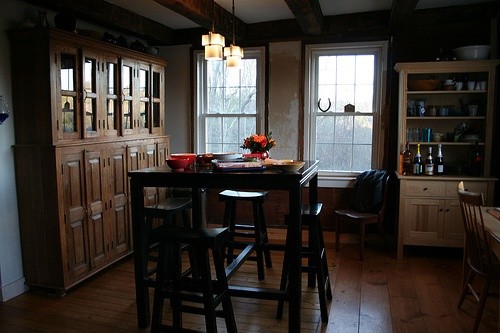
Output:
(127, 159), (320, 333)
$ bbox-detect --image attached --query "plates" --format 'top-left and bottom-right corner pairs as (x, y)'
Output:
(215, 159), (266, 171)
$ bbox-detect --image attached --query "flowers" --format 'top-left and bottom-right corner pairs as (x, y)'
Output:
(240, 132), (277, 153)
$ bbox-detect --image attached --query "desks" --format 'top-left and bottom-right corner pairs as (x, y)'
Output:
(461, 205), (500, 290)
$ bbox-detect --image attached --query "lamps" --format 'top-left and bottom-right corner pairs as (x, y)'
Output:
(201, 0), (244, 67)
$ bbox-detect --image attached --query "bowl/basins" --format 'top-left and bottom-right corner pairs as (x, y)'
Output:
(273, 161), (306, 173)
(453, 45), (491, 59)
(244, 157), (257, 162)
(243, 154), (261, 159)
(263, 162), (274, 170)
(212, 152), (242, 163)
(165, 158), (189, 171)
(196, 153), (213, 166)
(407, 78), (441, 91)
(171, 153), (196, 169)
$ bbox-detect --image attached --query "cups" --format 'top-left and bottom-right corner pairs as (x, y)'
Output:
(444, 77), (487, 91)
(408, 126), (434, 144)
(468, 105), (478, 117)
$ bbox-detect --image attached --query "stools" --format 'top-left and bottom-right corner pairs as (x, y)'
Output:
(143, 188), (238, 333)
(276, 202), (332, 322)
(219, 189), (273, 281)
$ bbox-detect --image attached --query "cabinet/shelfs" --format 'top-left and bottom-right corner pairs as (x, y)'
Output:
(394, 59), (500, 261)
(6, 25), (168, 295)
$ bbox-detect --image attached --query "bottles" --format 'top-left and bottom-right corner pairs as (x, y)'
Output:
(434, 145), (445, 176)
(412, 143), (424, 175)
(408, 97), (425, 116)
(400, 141), (413, 176)
(424, 147), (435, 176)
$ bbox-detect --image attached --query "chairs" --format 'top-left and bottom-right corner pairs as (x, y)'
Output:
(334, 174), (391, 261)
(456, 180), (500, 333)
